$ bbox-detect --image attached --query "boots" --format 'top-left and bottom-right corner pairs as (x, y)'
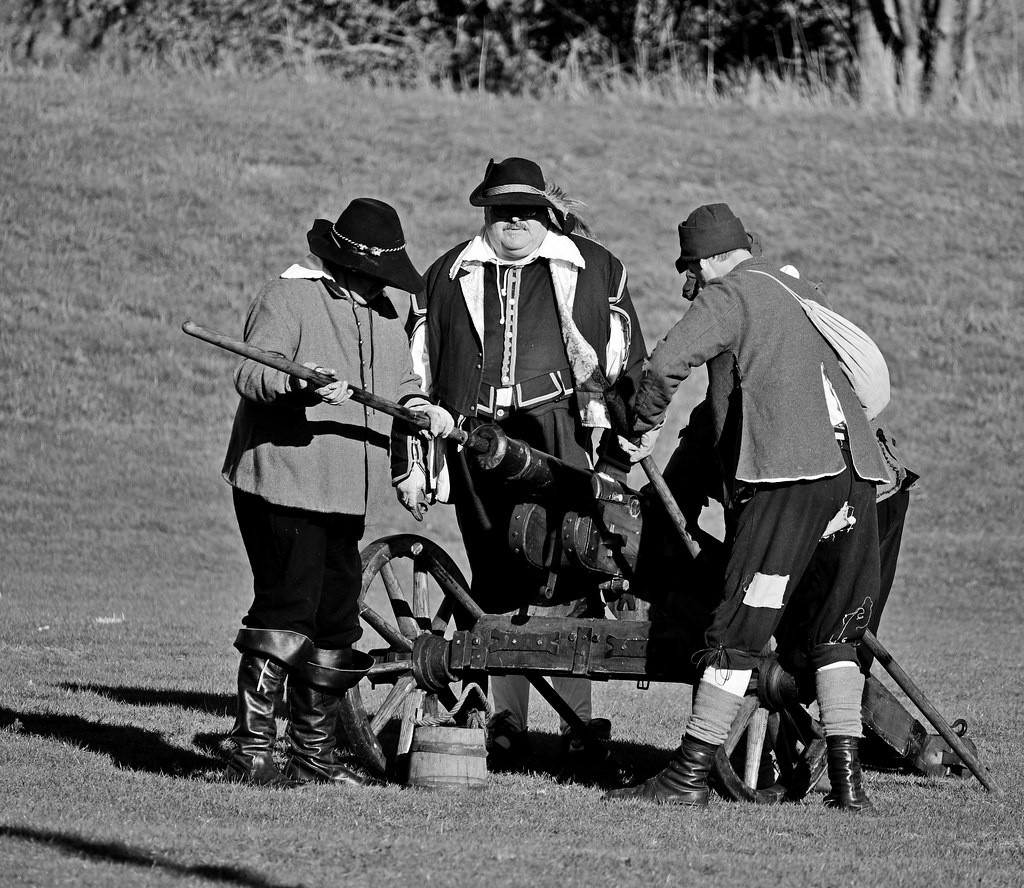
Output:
(226, 629), (303, 789)
(283, 649), (388, 788)
(824, 735), (873, 812)
(604, 733), (719, 809)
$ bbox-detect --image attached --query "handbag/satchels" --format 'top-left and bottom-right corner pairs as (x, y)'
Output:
(801, 299), (890, 422)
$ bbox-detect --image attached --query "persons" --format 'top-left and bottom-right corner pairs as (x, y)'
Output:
(390, 157), (667, 765)
(601, 204), (921, 816)
(223, 198), (455, 789)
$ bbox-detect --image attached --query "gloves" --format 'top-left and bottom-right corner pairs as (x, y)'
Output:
(411, 405), (454, 441)
(617, 414), (668, 463)
(311, 368), (354, 406)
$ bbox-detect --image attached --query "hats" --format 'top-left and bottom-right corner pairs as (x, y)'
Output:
(469, 157), (601, 242)
(306, 198), (425, 295)
(675, 203), (752, 273)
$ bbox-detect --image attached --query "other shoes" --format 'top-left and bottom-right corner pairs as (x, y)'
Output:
(488, 725), (535, 775)
(560, 728), (599, 784)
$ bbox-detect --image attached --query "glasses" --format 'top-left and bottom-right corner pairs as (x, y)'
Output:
(490, 206), (538, 217)
